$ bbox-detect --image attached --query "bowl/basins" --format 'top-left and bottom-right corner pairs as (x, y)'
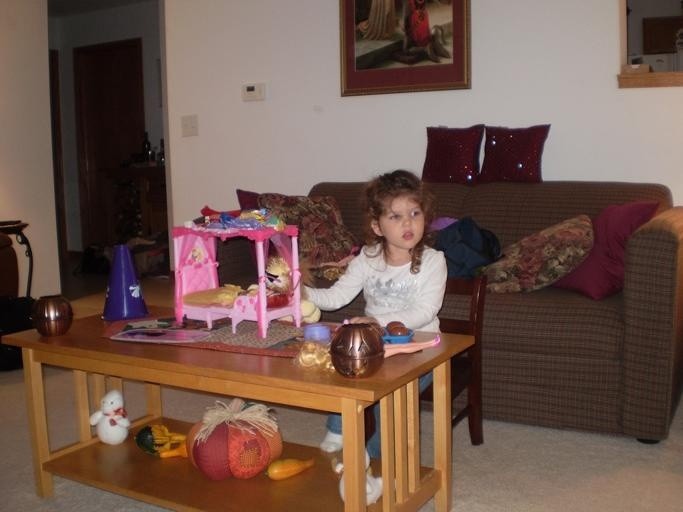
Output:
(130, 153), (145, 162)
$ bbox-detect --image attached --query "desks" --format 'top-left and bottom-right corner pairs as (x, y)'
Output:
(0, 304), (475, 511)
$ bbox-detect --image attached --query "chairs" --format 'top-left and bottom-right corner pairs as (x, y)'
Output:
(420, 273), (487, 444)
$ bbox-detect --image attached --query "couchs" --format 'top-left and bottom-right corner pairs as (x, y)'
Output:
(197, 181), (682, 445)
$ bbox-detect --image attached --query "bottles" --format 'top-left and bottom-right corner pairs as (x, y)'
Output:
(157, 139), (164, 167)
(143, 132), (150, 162)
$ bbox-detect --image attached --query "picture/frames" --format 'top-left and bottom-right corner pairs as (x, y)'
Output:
(339, 0), (470, 96)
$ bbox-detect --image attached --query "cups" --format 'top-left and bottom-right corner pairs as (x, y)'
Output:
(304, 323), (331, 343)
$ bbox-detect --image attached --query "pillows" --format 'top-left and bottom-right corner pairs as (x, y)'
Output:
(480, 123), (551, 184)
(552, 200), (660, 298)
(478, 213), (598, 294)
(421, 124), (485, 186)
(236, 188), (259, 211)
(259, 192), (365, 271)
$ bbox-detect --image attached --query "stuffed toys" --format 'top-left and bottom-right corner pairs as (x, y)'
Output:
(90, 390), (130, 445)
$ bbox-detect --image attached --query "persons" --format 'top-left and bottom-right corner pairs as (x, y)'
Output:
(293, 334), (441, 372)
(303, 169), (447, 463)
